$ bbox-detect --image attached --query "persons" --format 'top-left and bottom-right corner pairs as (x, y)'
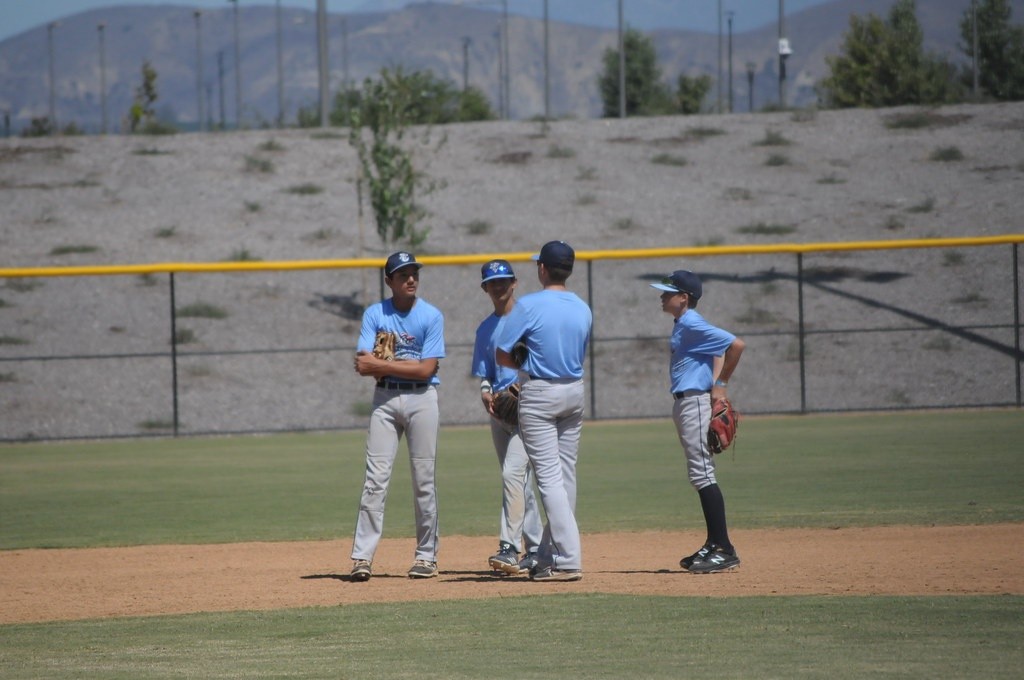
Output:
(351, 252), (446, 582)
(649, 270), (746, 574)
(471, 259), (543, 575)
(496, 240), (593, 582)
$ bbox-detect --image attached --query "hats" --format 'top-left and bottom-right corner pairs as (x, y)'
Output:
(481, 260), (515, 284)
(532, 240), (575, 273)
(649, 270), (702, 300)
(384, 252), (421, 277)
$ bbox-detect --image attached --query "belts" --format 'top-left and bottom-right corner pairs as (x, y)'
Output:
(377, 382), (426, 390)
(672, 390), (711, 401)
(529, 376), (551, 380)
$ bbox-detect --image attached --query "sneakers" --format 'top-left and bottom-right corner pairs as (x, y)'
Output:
(688, 546), (741, 573)
(529, 566), (583, 581)
(408, 561), (438, 578)
(351, 559), (371, 579)
(509, 553), (539, 575)
(488, 543), (520, 573)
(680, 541), (717, 569)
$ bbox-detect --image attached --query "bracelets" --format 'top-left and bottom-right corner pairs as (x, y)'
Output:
(715, 378), (728, 386)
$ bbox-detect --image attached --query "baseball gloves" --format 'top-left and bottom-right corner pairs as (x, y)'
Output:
(492, 382), (520, 426)
(511, 340), (528, 368)
(707, 396), (740, 453)
(373, 330), (397, 382)
